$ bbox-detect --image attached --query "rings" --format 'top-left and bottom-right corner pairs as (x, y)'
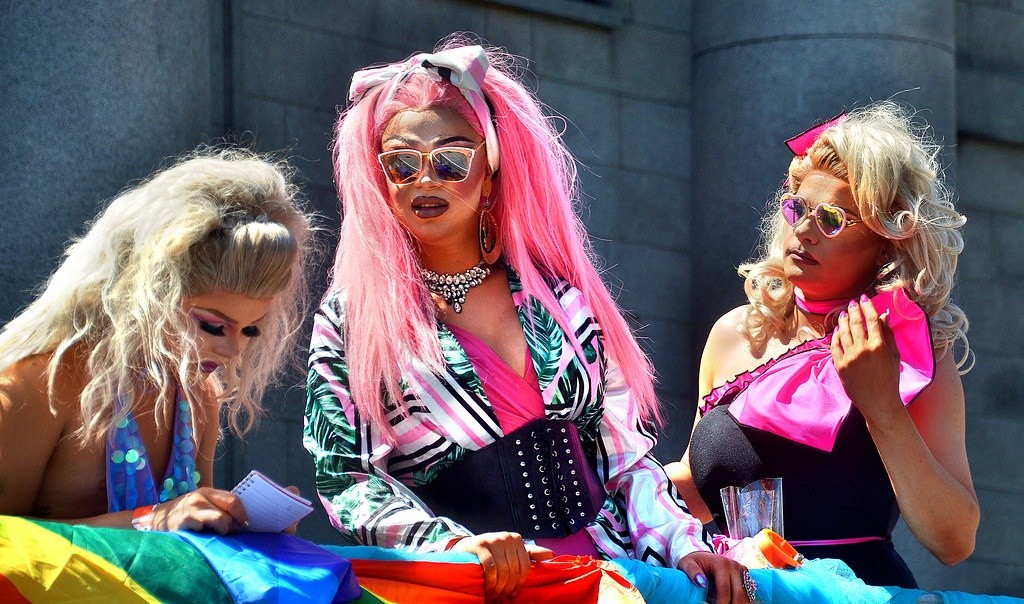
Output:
(743, 572), (757, 601)
(485, 563), (496, 570)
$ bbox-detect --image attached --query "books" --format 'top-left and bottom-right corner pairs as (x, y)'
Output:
(231, 471), (314, 533)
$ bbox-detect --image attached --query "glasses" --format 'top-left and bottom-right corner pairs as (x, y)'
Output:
(377, 140), (488, 187)
(778, 192), (863, 239)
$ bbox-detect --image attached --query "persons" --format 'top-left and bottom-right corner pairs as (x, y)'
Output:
(0, 154), (313, 535)
(662, 108), (981, 588)
(302, 42), (758, 604)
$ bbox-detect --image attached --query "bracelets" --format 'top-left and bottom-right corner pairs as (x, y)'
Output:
(132, 504), (159, 531)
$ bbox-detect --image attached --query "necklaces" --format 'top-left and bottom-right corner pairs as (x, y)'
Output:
(792, 287), (868, 315)
(421, 262), (491, 313)
